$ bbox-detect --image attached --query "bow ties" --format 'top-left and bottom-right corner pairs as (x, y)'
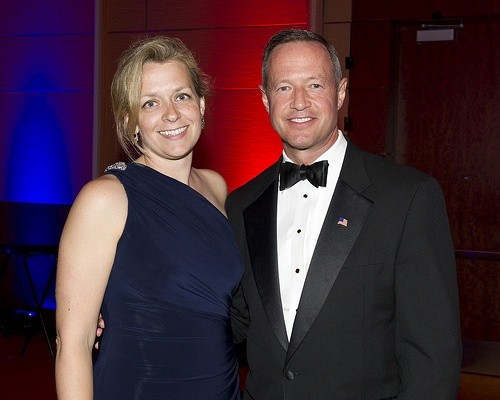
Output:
(280, 160), (328, 191)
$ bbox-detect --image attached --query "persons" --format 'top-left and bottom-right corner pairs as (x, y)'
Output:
(96, 29), (462, 400)
(56, 35), (245, 400)
